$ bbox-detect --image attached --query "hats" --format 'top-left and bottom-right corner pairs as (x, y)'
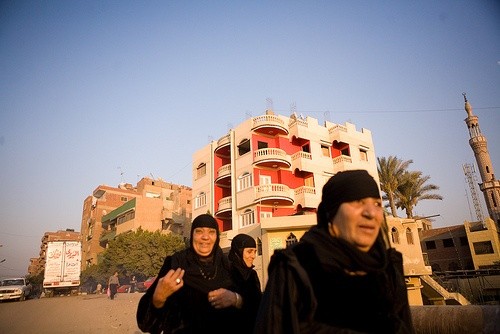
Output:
(322, 170), (380, 221)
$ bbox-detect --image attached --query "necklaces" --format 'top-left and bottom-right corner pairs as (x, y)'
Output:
(198, 267), (217, 280)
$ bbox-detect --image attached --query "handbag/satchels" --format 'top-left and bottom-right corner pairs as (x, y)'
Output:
(107, 288), (111, 297)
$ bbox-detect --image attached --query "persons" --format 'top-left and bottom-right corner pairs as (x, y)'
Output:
(136, 214), (258, 334)
(228, 234), (261, 294)
(108, 271), (120, 301)
(254, 169), (413, 334)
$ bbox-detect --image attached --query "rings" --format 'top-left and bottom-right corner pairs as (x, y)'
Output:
(176, 278), (181, 283)
(212, 297), (213, 300)
(214, 302), (215, 305)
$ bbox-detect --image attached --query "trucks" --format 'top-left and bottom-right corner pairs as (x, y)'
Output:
(43, 241), (82, 298)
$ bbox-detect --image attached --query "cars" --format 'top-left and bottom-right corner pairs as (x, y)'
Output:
(136, 277), (157, 292)
(118, 285), (133, 293)
(0, 278), (32, 302)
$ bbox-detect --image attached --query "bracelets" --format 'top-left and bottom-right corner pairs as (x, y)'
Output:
(236, 293), (241, 308)
